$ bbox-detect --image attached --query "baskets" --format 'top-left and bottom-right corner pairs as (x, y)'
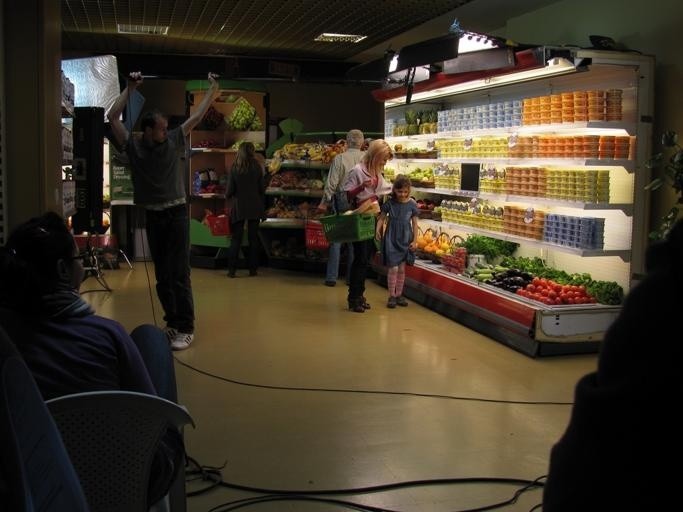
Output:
(319, 190), (376, 243)
(305, 208), (330, 249)
(417, 226), (465, 264)
(207, 197), (233, 236)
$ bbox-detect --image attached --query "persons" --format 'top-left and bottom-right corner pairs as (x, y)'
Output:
(376, 174), (419, 308)
(224, 142), (272, 278)
(4, 212), (183, 512)
(107, 71), (220, 351)
(345, 138), (417, 317)
(317, 129), (368, 287)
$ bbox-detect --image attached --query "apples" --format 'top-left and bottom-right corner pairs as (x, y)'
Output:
(411, 196), (439, 210)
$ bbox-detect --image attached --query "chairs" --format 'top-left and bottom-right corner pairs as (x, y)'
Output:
(46, 390), (195, 512)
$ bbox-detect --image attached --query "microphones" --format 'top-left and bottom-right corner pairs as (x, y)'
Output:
(406, 82), (414, 105)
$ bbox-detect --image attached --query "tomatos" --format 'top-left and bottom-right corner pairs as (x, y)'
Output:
(516, 277), (596, 305)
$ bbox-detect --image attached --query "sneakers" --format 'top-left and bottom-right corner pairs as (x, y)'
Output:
(227, 272), (235, 278)
(171, 332), (194, 350)
(387, 296), (397, 308)
(323, 280), (336, 286)
(164, 326), (177, 339)
(250, 271), (256, 276)
(397, 296), (408, 306)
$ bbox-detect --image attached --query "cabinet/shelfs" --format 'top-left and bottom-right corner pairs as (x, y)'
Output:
(189, 88), (272, 271)
(374, 49), (656, 357)
(267, 142), (373, 269)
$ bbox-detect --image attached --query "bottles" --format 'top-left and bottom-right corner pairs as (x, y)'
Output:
(387, 119), (394, 136)
(305, 149), (309, 157)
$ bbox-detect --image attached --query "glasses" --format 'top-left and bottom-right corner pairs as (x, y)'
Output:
(72, 250), (88, 262)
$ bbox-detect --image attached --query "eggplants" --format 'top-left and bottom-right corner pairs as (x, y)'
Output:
(485, 269), (536, 293)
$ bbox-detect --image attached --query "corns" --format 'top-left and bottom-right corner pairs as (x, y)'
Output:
(464, 264), (508, 282)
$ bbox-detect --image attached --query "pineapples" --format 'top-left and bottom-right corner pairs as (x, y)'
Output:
(391, 108), (436, 137)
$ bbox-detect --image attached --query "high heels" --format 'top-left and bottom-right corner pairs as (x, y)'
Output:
(348, 304), (365, 313)
(360, 296), (371, 309)
